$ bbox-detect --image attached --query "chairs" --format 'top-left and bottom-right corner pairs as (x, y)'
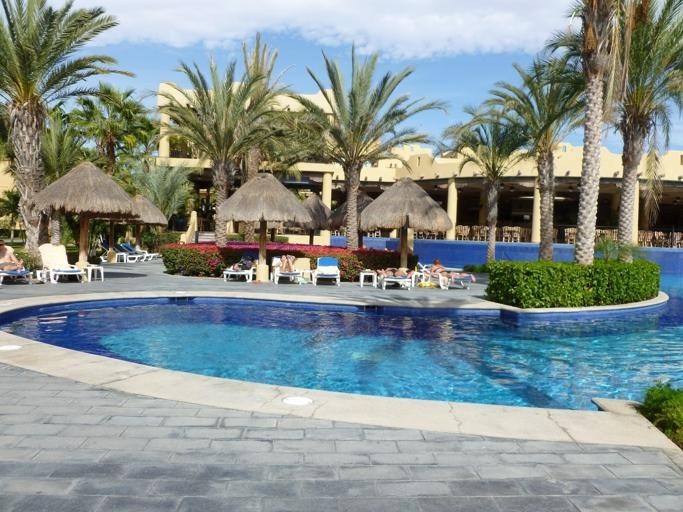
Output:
(1, 239), (106, 285)
(99, 240), (161, 263)
(223, 256), (478, 291)
(333, 223), (683, 249)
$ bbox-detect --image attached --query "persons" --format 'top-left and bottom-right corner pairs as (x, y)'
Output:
(431, 258), (476, 284)
(278, 254), (296, 272)
(376, 267), (413, 279)
(0, 239), (25, 272)
